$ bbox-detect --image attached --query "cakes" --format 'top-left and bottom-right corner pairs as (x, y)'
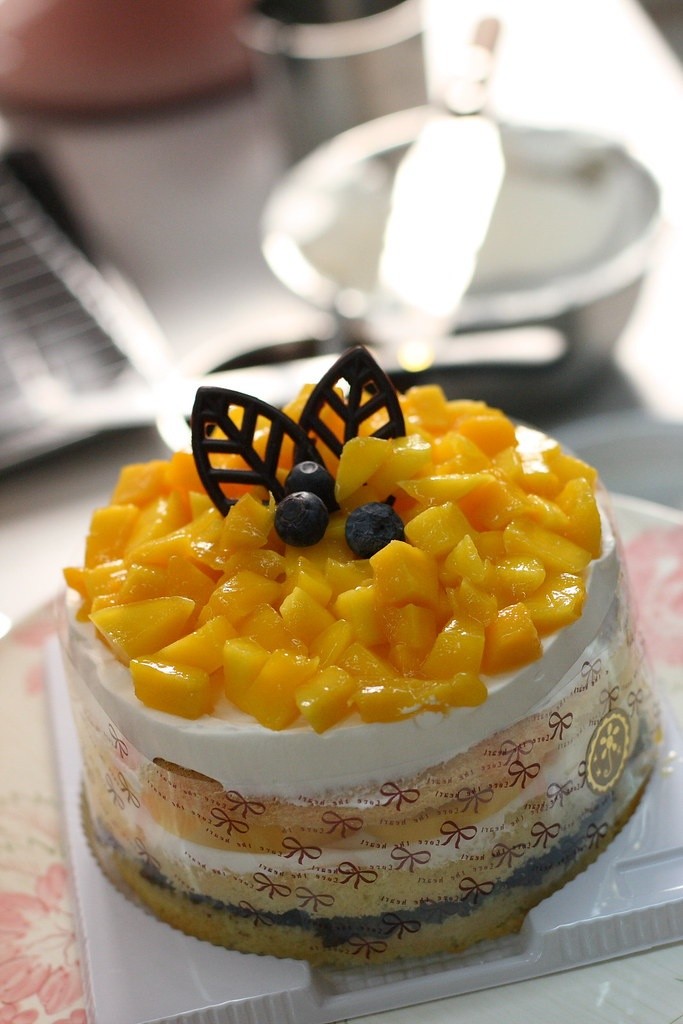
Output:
(57, 347), (660, 968)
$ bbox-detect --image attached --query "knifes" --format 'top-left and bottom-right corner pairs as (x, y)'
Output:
(375, 16), (506, 341)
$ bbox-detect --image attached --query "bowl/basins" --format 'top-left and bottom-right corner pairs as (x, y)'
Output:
(260, 100), (665, 416)
(0, 0), (250, 114)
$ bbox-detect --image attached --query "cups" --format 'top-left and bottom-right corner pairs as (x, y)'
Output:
(233, 0), (429, 167)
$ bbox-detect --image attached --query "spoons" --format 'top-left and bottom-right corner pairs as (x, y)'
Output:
(155, 326), (567, 456)
